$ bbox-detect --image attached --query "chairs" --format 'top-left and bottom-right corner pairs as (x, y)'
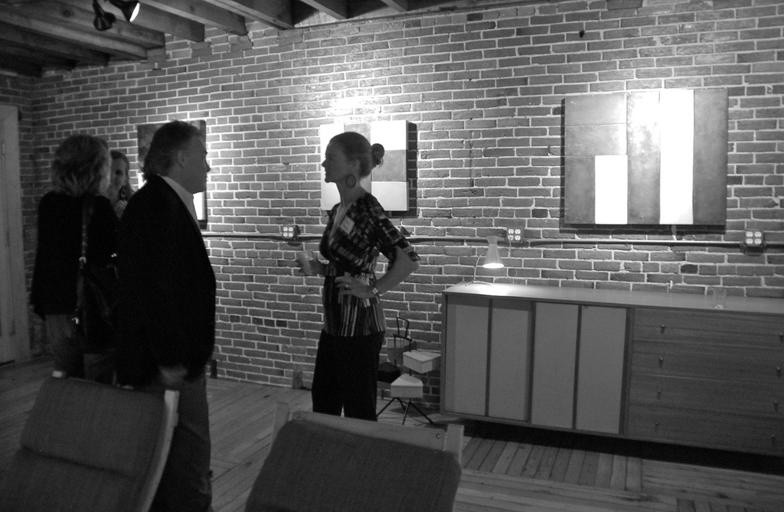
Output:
(243, 404), (465, 511)
(0, 370), (180, 512)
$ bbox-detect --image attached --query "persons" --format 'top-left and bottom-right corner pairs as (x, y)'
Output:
(295, 132), (419, 420)
(107, 121), (216, 512)
(30, 133), (121, 384)
(109, 150), (132, 220)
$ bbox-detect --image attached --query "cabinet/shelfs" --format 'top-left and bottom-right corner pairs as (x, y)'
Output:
(442, 290), (531, 430)
(621, 306), (784, 460)
(531, 300), (629, 438)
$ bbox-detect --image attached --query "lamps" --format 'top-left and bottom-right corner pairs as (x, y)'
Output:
(90, 0), (117, 31)
(464, 236), (504, 289)
(107, 0), (141, 24)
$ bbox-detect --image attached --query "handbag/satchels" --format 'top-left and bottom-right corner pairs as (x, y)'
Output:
(72, 263), (124, 355)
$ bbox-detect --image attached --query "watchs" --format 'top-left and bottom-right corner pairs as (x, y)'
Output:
(371, 282), (383, 298)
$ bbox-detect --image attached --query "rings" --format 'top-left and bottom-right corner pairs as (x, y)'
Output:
(345, 283), (349, 289)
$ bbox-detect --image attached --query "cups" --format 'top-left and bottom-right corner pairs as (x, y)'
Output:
(297, 251), (317, 277)
